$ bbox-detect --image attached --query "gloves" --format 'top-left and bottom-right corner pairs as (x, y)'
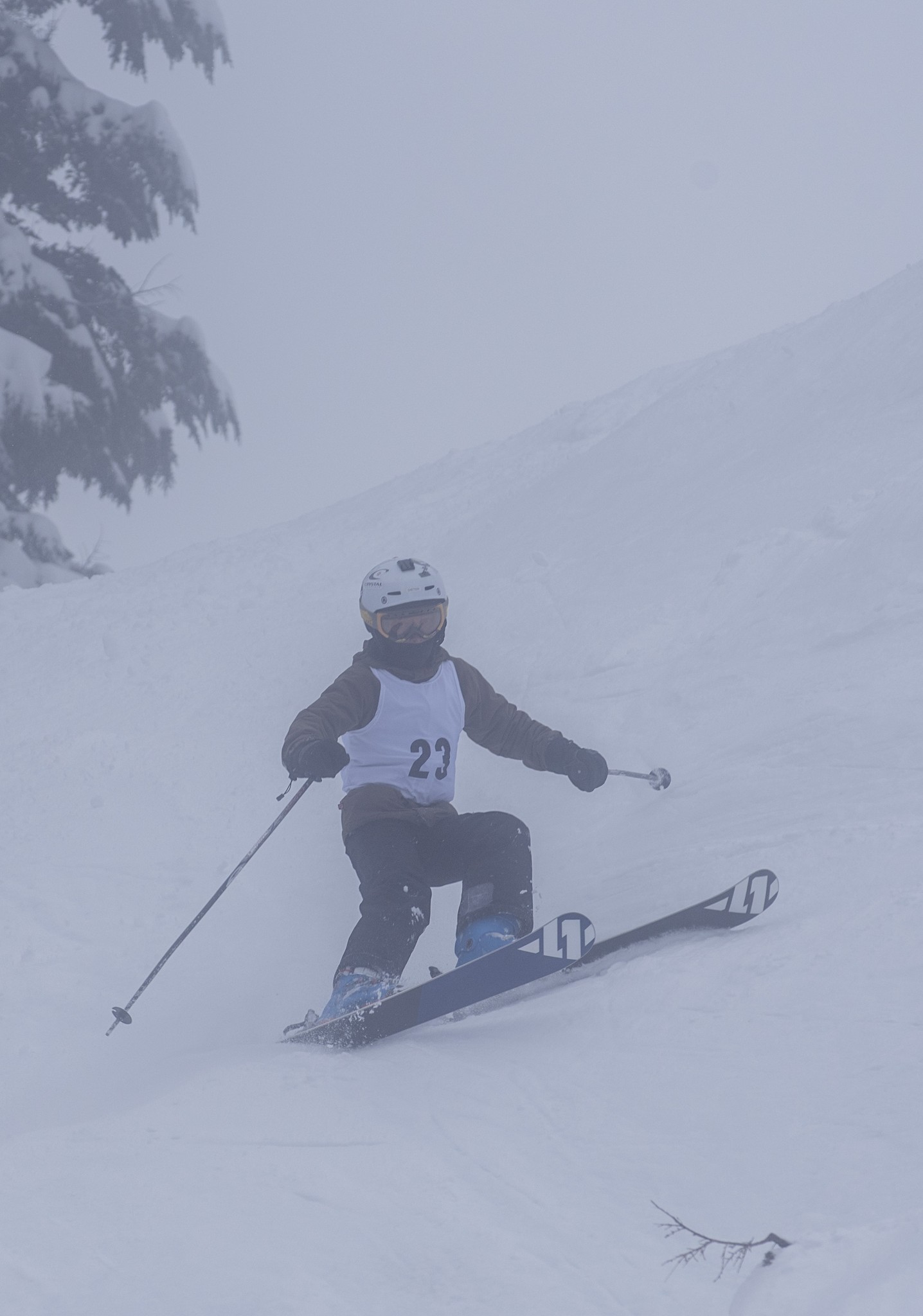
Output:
(292, 738), (350, 778)
(543, 737), (607, 792)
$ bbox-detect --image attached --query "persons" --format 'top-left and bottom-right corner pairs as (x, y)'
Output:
(281, 554), (609, 1022)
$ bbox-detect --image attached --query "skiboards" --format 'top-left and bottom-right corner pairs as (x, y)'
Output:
(276, 868), (780, 1051)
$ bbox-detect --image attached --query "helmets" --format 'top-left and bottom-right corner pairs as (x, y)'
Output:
(358, 557), (448, 660)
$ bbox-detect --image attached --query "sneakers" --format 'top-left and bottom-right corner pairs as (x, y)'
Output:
(453, 914), (520, 969)
(315, 966), (403, 1028)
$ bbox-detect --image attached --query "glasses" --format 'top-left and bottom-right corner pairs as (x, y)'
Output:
(376, 603), (445, 643)
